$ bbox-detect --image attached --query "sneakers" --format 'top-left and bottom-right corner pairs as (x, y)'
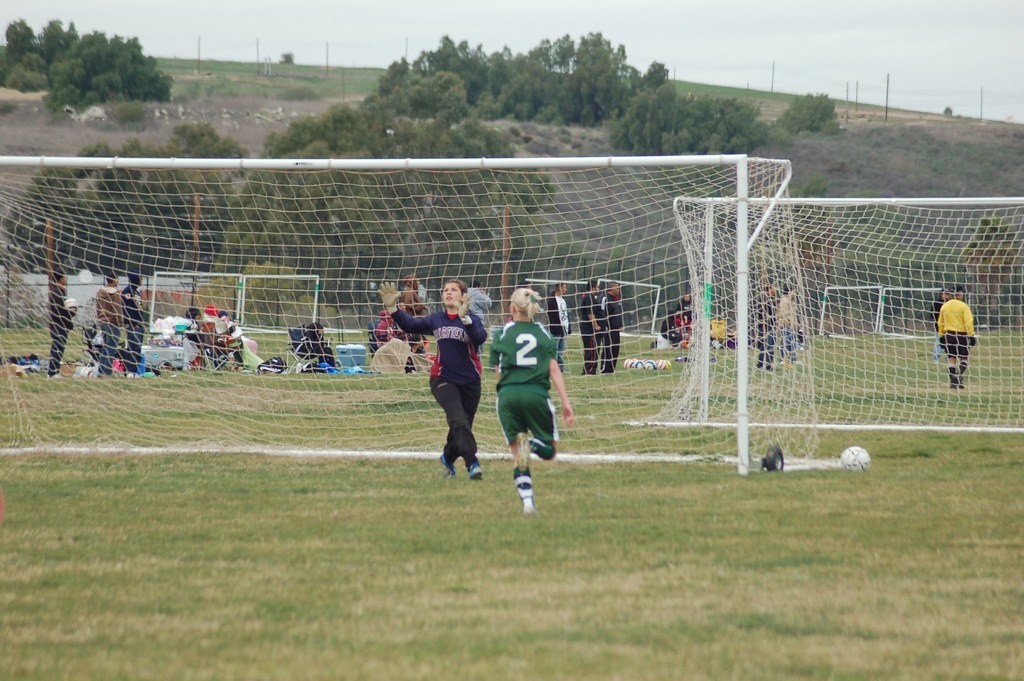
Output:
(439, 453), (456, 478)
(468, 463), (482, 479)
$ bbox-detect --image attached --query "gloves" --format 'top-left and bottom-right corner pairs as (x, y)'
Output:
(968, 336), (976, 346)
(377, 282), (402, 314)
(453, 292), (471, 319)
(939, 336), (946, 348)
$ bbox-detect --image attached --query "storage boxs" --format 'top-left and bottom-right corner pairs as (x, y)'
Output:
(335, 344), (366, 367)
(143, 347), (184, 369)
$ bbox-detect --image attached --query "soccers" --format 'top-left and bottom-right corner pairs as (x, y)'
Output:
(838, 446), (871, 473)
(623, 357), (670, 370)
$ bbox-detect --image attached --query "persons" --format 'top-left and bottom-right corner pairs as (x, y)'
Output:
(378, 279), (488, 480)
(489, 289), (573, 514)
(376, 272), (492, 372)
(47, 268), (73, 378)
(757, 276), (804, 373)
(121, 269), (145, 379)
(301, 322), (335, 373)
(930, 284), (976, 389)
(661, 293), (692, 347)
(546, 280), (625, 376)
(185, 305), (246, 371)
(96, 269), (125, 378)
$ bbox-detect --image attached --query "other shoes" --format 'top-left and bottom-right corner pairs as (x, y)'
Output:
(523, 511), (539, 519)
(516, 432), (530, 473)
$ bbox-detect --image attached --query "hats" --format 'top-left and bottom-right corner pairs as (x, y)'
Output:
(940, 288), (954, 293)
(953, 284), (966, 293)
(206, 305), (219, 317)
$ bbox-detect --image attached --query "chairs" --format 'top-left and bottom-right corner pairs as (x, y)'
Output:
(366, 321), (380, 359)
(286, 324), (334, 374)
(80, 324), (126, 366)
(201, 321), (244, 372)
